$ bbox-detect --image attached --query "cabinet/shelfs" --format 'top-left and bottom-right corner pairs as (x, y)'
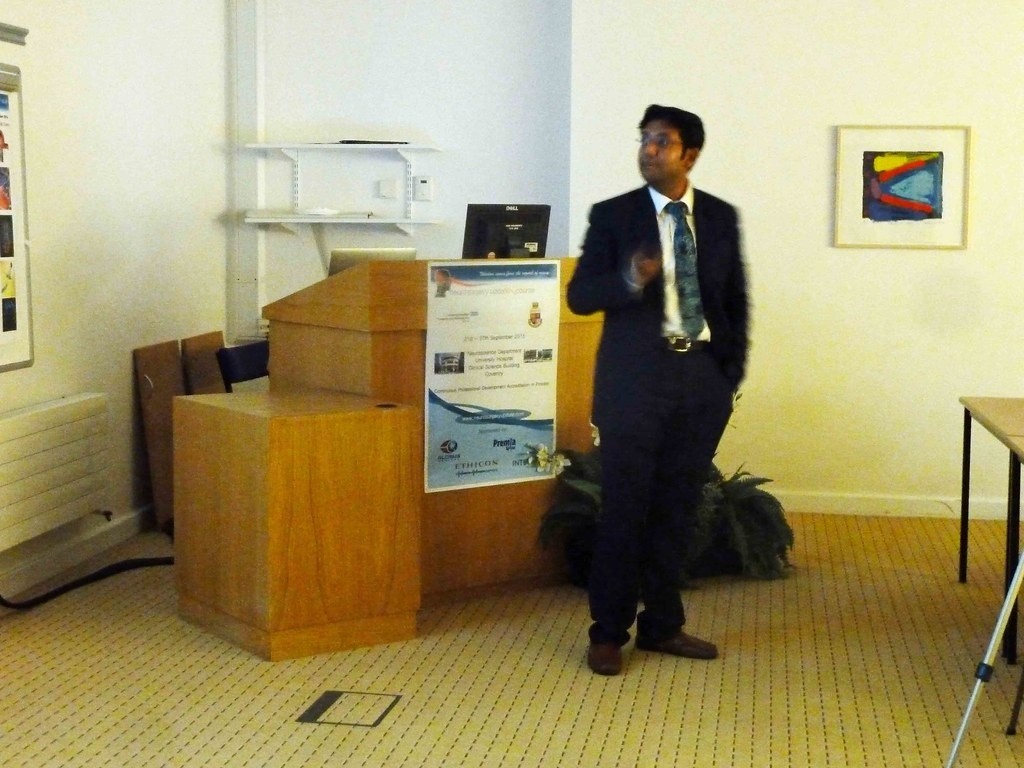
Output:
(244, 143), (442, 237)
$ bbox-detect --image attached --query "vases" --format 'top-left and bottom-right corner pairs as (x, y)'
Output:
(561, 539), (598, 588)
(693, 545), (742, 577)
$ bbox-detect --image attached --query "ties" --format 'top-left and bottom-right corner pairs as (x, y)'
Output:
(664, 201), (705, 339)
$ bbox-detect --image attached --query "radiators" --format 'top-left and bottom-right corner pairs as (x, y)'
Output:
(0, 392), (117, 551)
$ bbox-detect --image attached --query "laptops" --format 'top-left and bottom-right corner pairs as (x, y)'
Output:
(328, 248), (416, 277)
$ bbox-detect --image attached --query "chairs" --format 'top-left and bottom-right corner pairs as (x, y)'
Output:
(216, 340), (270, 391)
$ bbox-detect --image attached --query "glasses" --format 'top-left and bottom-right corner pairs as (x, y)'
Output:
(637, 135), (685, 149)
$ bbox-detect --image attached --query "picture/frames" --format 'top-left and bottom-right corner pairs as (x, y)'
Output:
(833, 124), (973, 253)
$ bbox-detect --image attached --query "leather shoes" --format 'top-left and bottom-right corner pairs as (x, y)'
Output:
(587, 641), (623, 675)
(637, 630), (718, 659)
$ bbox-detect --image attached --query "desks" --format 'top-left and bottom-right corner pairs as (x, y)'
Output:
(958, 395), (1024, 664)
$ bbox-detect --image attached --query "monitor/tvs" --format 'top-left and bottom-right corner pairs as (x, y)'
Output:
(462, 204), (551, 259)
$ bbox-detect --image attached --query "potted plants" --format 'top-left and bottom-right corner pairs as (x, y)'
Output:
(681, 460), (795, 581)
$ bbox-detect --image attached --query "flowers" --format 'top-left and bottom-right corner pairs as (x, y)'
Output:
(522, 417), (605, 548)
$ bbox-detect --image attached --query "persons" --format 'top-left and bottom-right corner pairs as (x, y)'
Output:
(566, 103), (749, 677)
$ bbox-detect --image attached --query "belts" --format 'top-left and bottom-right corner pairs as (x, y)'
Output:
(662, 336), (709, 352)
